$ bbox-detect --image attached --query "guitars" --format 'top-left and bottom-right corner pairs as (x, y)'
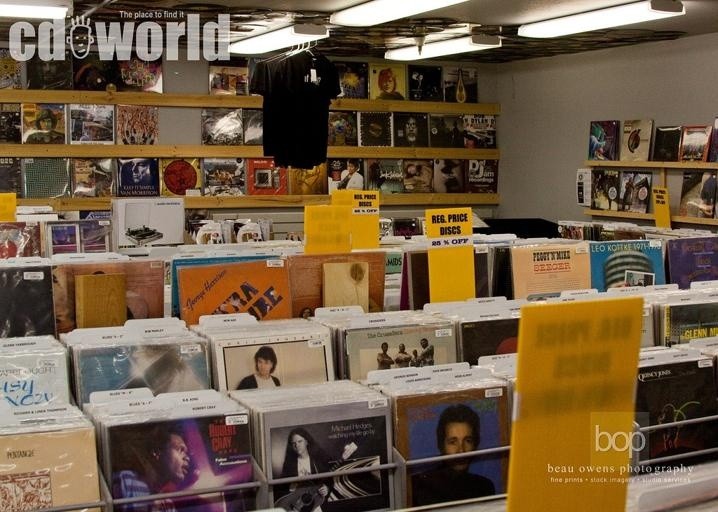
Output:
(274, 442), (358, 512)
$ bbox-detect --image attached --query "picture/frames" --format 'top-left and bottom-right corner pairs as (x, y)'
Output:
(678, 123), (713, 162)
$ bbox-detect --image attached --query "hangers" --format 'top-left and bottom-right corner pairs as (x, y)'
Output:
(253, 38), (321, 63)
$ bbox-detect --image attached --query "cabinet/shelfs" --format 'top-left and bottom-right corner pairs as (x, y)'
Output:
(0, 88), (502, 213)
(584, 159), (718, 225)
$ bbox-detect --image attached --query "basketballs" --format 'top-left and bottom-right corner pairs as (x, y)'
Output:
(164, 160), (197, 195)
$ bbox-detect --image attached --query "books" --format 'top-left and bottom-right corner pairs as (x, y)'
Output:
(0, 50), (718, 512)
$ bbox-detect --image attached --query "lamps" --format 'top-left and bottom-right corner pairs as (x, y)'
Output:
(384, 23), (503, 62)
(329, 0), (472, 28)
(228, 23), (330, 55)
(0, 0), (75, 20)
(517, 0), (687, 40)
